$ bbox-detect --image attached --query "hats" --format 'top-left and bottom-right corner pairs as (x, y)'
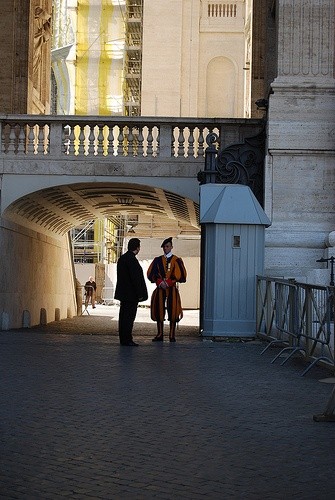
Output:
(161, 237), (173, 247)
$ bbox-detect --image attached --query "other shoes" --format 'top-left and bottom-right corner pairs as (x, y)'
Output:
(122, 340), (139, 347)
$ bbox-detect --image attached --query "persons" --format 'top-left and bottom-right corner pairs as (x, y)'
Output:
(147, 237), (187, 342)
(114, 237), (148, 346)
(84, 276), (96, 309)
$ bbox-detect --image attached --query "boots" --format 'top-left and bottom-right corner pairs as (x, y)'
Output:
(152, 322), (163, 342)
(169, 321), (177, 343)
